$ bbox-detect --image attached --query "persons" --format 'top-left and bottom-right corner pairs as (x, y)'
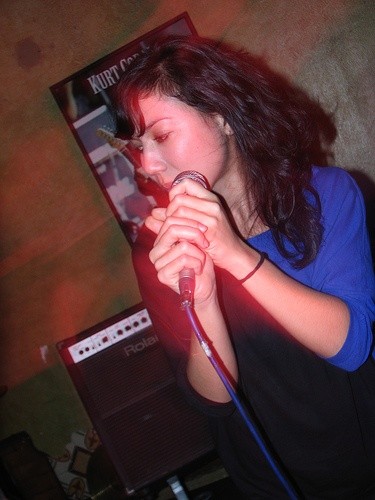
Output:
(112, 39), (375, 500)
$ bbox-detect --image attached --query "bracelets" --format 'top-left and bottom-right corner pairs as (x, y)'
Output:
(241, 253), (265, 281)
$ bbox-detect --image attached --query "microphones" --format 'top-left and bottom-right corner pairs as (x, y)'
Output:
(167, 170), (208, 295)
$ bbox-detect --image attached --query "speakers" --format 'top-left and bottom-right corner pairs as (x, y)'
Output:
(59, 302), (213, 495)
